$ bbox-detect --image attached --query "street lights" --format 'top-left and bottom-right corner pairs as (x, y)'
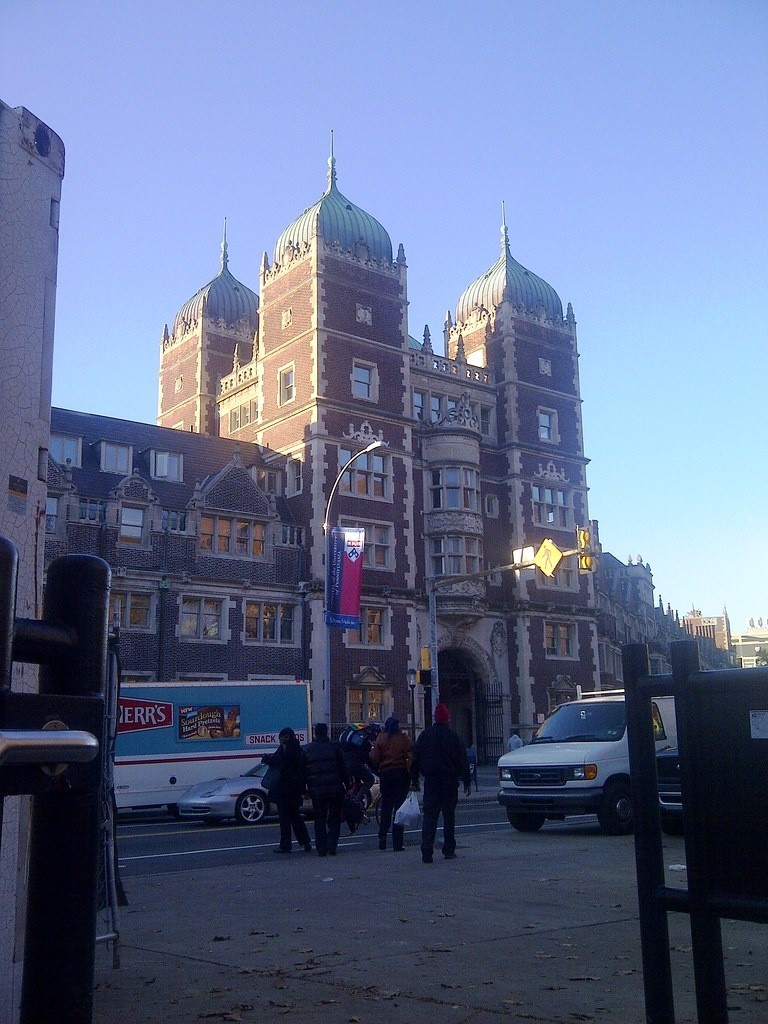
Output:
(324, 441), (388, 740)
(408, 674), (421, 792)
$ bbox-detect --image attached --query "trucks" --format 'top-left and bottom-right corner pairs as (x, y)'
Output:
(114, 681), (312, 815)
(497, 685), (679, 836)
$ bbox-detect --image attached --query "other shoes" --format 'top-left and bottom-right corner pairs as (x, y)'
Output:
(441, 852), (457, 859)
(393, 847), (406, 852)
(378, 839), (386, 850)
(423, 855), (433, 864)
(328, 849), (338, 855)
(273, 846), (292, 854)
(301, 841), (312, 850)
(353, 797), (364, 811)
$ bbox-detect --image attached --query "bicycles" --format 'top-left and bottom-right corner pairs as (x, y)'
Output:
(332, 778), (396, 837)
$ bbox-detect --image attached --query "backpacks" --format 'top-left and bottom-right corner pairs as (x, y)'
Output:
(339, 721), (372, 748)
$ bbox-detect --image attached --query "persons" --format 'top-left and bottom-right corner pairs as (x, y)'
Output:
(260, 728), (311, 853)
(414, 703), (472, 864)
(532, 728), (538, 739)
(301, 723), (351, 856)
(339, 723), (383, 833)
(506, 730), (523, 752)
(371, 718), (421, 852)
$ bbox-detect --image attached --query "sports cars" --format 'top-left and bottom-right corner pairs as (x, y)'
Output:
(177, 756), (317, 825)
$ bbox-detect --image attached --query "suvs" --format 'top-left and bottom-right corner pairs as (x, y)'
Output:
(654, 747), (682, 835)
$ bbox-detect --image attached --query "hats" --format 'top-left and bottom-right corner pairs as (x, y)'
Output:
(434, 703), (450, 723)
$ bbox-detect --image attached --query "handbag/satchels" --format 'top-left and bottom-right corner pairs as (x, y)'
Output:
(260, 764), (281, 789)
(394, 791), (423, 829)
